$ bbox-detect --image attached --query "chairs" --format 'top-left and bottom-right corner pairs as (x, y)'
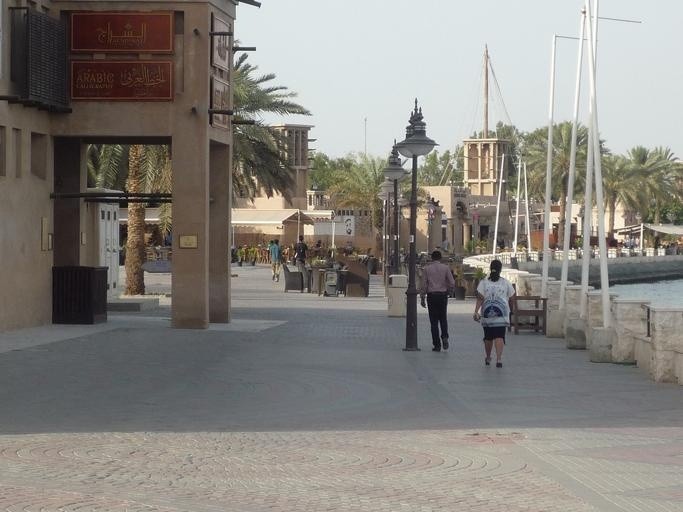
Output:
(507, 284), (549, 335)
(282, 248), (378, 298)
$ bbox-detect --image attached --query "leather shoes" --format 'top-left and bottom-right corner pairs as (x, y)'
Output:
(443, 337), (448, 349)
(432, 346), (441, 351)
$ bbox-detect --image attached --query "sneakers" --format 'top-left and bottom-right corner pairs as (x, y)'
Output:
(486, 359), (490, 365)
(496, 363), (502, 368)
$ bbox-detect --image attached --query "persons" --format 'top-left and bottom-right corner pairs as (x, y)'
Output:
(247, 243), (257, 265)
(236, 245), (244, 266)
(365, 247), (374, 257)
(471, 258), (516, 367)
(270, 238), (282, 282)
(399, 247), (406, 262)
(419, 250), (455, 352)
(293, 234), (307, 267)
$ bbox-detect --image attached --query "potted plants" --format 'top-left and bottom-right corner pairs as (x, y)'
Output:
(454, 265), (468, 300)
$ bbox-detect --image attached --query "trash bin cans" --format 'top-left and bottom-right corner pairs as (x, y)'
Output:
(322, 268), (339, 297)
(386, 274), (408, 318)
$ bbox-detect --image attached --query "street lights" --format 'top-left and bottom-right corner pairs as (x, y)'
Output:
(395, 97), (440, 351)
(378, 177), (395, 283)
(382, 139), (405, 275)
